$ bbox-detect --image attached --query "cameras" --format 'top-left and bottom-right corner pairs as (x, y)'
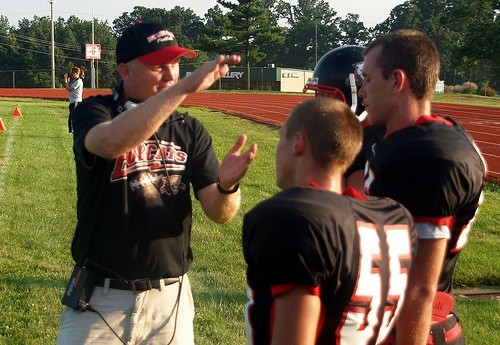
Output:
(61, 73), (71, 78)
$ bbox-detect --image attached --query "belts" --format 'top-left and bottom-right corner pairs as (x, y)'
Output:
(95, 277), (179, 294)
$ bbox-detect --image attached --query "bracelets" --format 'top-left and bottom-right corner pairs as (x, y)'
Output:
(216, 177), (239, 194)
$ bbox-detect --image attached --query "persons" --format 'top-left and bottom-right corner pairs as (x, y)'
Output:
(242, 98), (419, 345)
(64, 67), (84, 133)
(357, 29), (485, 345)
(57, 20), (258, 345)
(302, 44), (383, 193)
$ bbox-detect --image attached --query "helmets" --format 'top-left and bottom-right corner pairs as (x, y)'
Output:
(303, 45), (369, 128)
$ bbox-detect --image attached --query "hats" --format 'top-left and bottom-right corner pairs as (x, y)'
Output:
(116, 19), (197, 65)
